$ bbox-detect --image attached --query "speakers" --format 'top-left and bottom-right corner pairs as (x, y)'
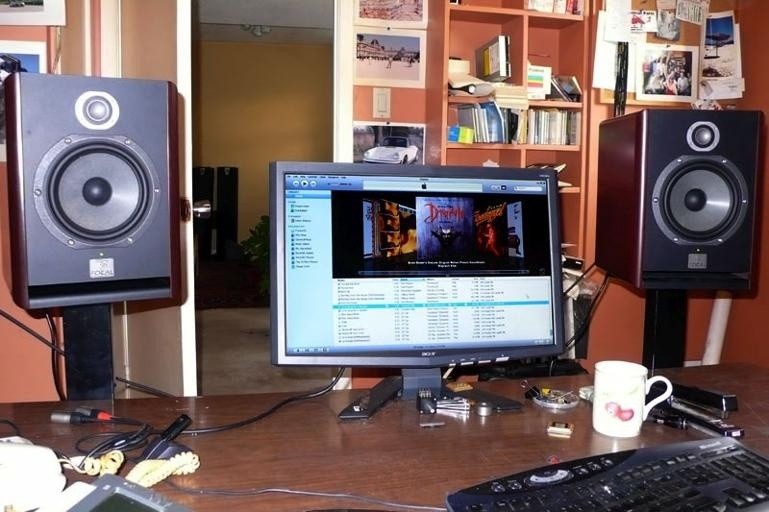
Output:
(2, 71), (179, 311)
(217, 166), (238, 257)
(594, 108), (765, 300)
(193, 166), (214, 255)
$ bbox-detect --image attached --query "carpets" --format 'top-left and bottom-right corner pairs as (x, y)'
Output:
(196, 254), (269, 311)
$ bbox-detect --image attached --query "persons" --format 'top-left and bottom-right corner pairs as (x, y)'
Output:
(643, 51), (691, 95)
(358, 51), (421, 71)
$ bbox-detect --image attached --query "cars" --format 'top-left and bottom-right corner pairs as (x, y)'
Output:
(363, 136), (419, 165)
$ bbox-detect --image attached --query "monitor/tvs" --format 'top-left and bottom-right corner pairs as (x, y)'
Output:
(66, 472), (193, 512)
(269, 160), (566, 418)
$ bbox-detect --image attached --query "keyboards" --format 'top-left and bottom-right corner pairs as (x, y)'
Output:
(445, 436), (769, 511)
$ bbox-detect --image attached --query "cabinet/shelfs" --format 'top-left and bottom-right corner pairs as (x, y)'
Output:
(424, 0), (596, 281)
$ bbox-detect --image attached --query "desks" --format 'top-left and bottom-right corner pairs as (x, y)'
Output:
(1, 359), (769, 510)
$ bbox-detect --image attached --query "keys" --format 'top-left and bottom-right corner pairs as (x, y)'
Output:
(416, 388), (472, 416)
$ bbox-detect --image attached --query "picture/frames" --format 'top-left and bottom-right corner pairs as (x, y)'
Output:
(633, 41), (699, 102)
(348, 1), (430, 90)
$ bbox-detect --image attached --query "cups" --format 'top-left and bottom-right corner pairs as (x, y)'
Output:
(591, 359), (674, 442)
